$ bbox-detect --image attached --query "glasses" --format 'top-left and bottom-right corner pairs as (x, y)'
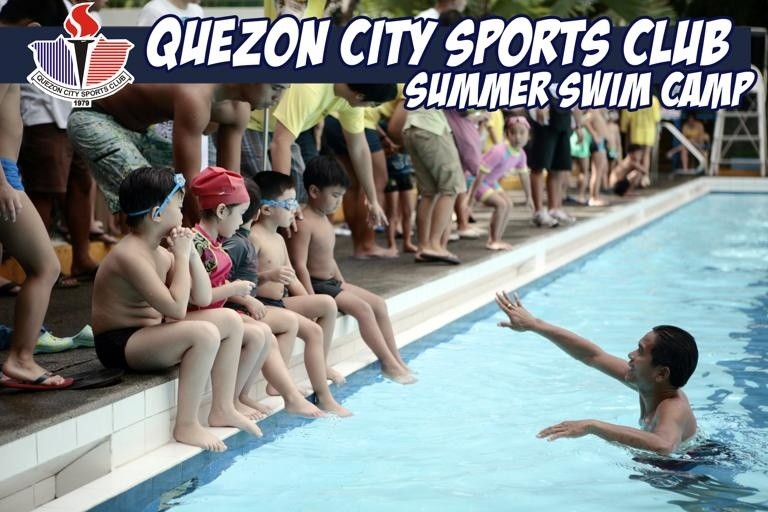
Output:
(506, 116), (529, 128)
(260, 197), (297, 211)
(151, 173), (187, 221)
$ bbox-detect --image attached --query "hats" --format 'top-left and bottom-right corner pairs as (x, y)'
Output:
(188, 166), (250, 213)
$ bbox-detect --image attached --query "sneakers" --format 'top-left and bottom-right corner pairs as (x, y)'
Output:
(549, 209), (576, 226)
(532, 211), (558, 228)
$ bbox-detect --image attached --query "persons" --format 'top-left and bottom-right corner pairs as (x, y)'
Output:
(1, 2), (744, 487)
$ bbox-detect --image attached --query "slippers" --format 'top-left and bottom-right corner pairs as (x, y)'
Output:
(0, 282), (22, 297)
(0, 369), (75, 391)
(52, 272), (80, 291)
(73, 325), (97, 347)
(414, 253), (443, 263)
(31, 330), (75, 355)
(419, 250), (462, 265)
